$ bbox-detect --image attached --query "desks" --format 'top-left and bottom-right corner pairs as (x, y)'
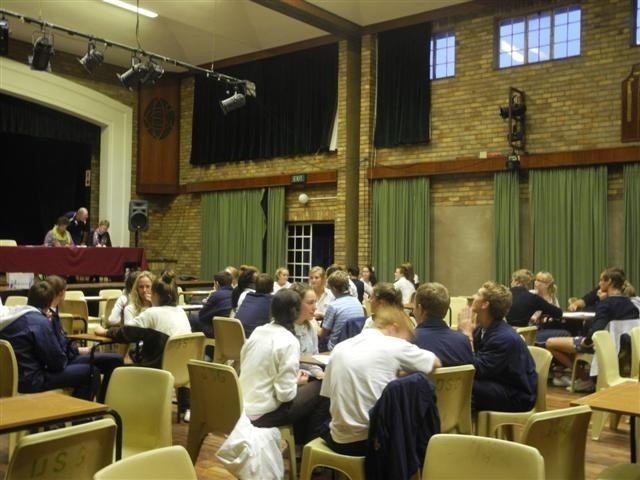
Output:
(1, 247), (145, 284)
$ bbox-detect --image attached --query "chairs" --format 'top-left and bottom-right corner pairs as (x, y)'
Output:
(0, 239), (17, 247)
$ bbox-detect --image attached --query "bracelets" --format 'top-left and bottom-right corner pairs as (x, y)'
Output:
(81, 241), (87, 244)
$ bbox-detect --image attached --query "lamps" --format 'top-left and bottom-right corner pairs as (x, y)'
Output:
(1, 0), (262, 115)
(499, 95), (527, 169)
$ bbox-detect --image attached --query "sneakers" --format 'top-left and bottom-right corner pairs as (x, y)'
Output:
(552, 374), (582, 386)
(565, 377), (595, 393)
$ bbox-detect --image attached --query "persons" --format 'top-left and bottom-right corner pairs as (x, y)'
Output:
(43, 216), (75, 248)
(86, 220), (112, 282)
(65, 208), (89, 284)
(1, 263), (638, 480)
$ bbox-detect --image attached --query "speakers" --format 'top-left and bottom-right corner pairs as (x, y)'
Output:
(128, 199), (149, 232)
